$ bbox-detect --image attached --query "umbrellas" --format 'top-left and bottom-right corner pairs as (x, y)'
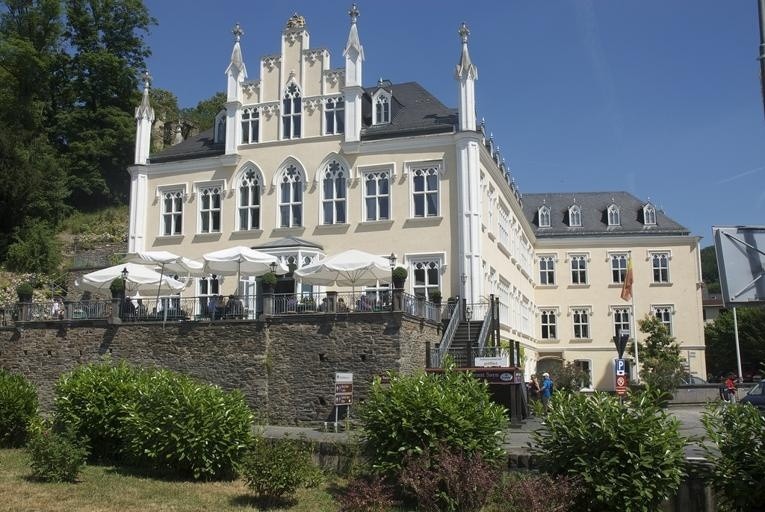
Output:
(201, 246), (289, 296)
(120, 251), (210, 318)
(74, 261), (185, 318)
(293, 250), (408, 312)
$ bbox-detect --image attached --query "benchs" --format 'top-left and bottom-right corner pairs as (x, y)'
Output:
(124, 309), (247, 322)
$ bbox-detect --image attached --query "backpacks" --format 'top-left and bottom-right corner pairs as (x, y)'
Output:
(549, 383), (553, 395)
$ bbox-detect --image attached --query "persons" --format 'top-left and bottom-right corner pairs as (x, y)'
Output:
(536, 372), (553, 425)
(719, 378), (737, 426)
(726, 375), (737, 412)
(525, 374), (540, 415)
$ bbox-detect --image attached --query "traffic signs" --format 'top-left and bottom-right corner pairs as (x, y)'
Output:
(335, 372), (353, 384)
(334, 395), (354, 406)
(335, 384), (355, 395)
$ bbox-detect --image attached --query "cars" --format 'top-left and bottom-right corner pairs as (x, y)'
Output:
(740, 379), (764, 416)
(667, 373), (708, 384)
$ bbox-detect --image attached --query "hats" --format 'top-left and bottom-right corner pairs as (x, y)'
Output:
(543, 373), (549, 377)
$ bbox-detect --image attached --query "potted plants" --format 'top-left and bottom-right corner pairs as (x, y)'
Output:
(391, 265), (408, 288)
(259, 271), (277, 293)
(109, 277), (126, 298)
(14, 280), (34, 302)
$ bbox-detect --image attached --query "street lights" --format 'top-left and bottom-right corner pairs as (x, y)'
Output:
(389, 252), (397, 312)
(269, 261), (277, 274)
(460, 271), (467, 322)
(122, 267), (129, 313)
(464, 307), (476, 368)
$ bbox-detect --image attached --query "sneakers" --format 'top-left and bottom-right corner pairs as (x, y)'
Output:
(541, 422), (546, 425)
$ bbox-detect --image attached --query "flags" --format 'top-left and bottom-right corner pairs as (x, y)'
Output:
(620, 255), (633, 302)
